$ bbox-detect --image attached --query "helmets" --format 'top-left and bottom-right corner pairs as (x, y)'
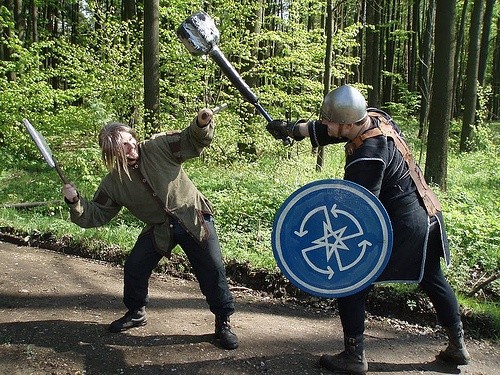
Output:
(321, 86), (367, 125)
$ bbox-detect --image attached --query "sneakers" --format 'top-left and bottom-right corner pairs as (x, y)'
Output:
(109, 310), (148, 333)
(215, 323), (238, 350)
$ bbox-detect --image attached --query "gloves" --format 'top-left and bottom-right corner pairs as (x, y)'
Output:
(265, 118), (307, 141)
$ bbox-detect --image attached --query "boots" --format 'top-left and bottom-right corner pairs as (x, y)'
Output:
(438, 323), (470, 365)
(320, 333), (368, 375)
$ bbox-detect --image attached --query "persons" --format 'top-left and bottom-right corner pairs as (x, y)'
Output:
(64, 106), (238, 350)
(267, 85), (471, 375)
